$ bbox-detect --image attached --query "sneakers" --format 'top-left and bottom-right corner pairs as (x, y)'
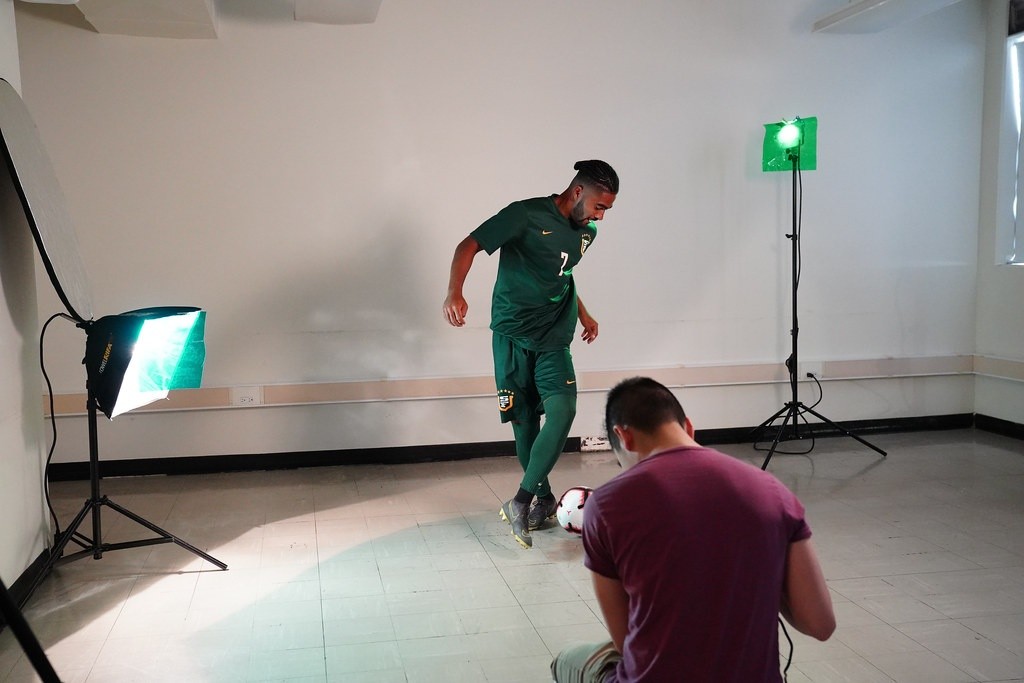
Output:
(528, 496), (558, 531)
(499, 499), (533, 549)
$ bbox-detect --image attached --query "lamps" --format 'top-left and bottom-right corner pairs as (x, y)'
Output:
(776, 116), (806, 162)
(82, 306), (200, 419)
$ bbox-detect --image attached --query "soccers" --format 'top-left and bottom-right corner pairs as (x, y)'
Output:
(555, 484), (595, 537)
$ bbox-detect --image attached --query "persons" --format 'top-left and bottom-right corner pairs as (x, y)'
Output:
(550, 377), (836, 683)
(442, 159), (619, 548)
